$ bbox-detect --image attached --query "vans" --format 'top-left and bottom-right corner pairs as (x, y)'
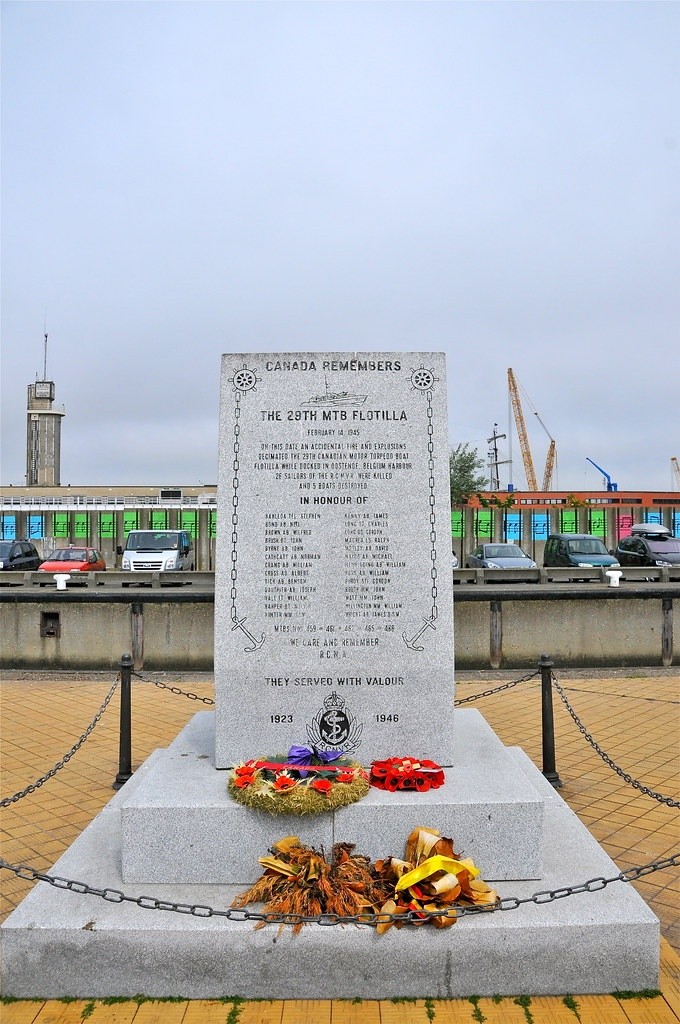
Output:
(116, 529), (195, 588)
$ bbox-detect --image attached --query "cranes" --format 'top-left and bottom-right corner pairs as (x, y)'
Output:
(507, 368), (557, 491)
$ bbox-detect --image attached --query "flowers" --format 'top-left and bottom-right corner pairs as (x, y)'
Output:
(230, 745), (368, 814)
(371, 757), (444, 793)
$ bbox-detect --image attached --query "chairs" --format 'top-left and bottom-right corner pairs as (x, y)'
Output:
(63, 553), (70, 559)
(497, 548), (511, 556)
(158, 536), (177, 547)
(569, 546), (574, 552)
(82, 554), (86, 558)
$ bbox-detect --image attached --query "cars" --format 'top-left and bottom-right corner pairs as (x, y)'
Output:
(466, 542), (540, 584)
(615, 527), (680, 582)
(452, 552), (460, 584)
(543, 536), (622, 582)
(38, 548), (106, 587)
(0, 539), (42, 586)
